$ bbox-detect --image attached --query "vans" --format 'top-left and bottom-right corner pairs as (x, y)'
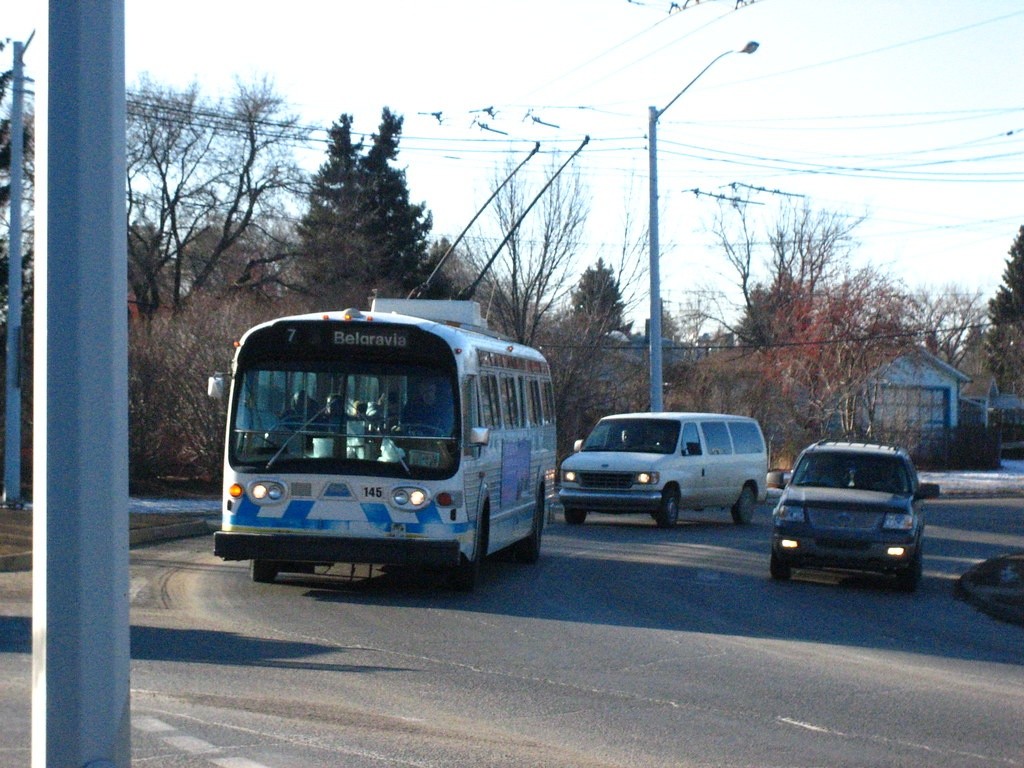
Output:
(559, 412), (768, 528)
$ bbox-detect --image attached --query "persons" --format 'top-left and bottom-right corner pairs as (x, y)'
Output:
(615, 426), (639, 450)
(279, 371), (455, 436)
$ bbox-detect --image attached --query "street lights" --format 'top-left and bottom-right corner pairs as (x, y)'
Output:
(649, 40), (762, 415)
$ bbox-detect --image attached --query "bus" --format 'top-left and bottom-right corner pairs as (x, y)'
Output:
(207, 298), (557, 583)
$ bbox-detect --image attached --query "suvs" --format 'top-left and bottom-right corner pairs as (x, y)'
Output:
(767, 438), (940, 590)
